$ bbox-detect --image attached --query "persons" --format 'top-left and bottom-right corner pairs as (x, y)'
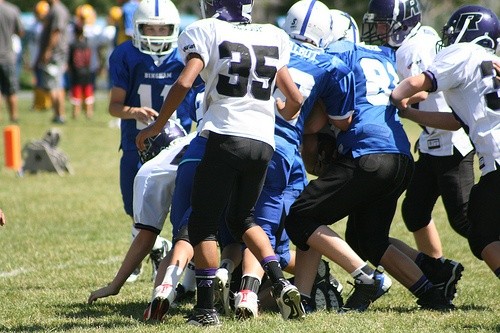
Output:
(135, 0), (306, 325)
(391, 5), (500, 283)
(208, 0), (355, 317)
(110, 0), (206, 300)
(360, 0), (482, 256)
(0, 1), (137, 124)
(287, 7), (464, 311)
(86, 115), (393, 313)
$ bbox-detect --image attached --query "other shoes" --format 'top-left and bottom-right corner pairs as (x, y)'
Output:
(53, 117), (66, 123)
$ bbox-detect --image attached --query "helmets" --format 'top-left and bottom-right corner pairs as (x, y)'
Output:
(202, 0), (255, 21)
(331, 9), (360, 42)
(285, 0), (331, 49)
(445, 4), (499, 53)
(35, 1), (122, 23)
(366, 0), (421, 47)
(134, 0), (179, 46)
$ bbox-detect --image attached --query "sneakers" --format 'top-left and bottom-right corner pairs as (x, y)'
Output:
(124, 239), (391, 327)
(433, 258), (463, 302)
(416, 289), (456, 311)
